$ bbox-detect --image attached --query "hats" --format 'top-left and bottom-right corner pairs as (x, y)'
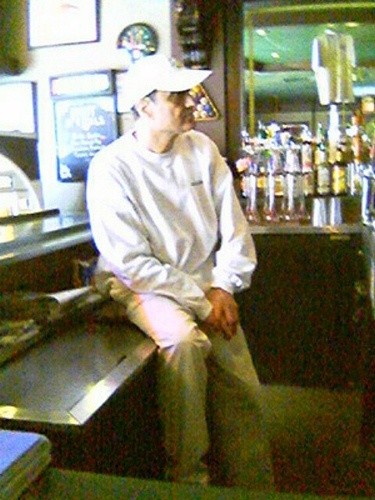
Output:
(124, 54), (213, 111)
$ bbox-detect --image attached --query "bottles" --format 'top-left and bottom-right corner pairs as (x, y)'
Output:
(243, 152), (257, 199)
(333, 142), (349, 196)
(257, 154), (269, 199)
(301, 141), (315, 195)
(273, 151), (287, 196)
(318, 143), (329, 196)
(287, 153), (301, 194)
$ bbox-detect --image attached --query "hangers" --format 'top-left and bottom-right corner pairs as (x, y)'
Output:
(324, 28), (337, 36)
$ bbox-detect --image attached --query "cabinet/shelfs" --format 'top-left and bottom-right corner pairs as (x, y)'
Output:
(0, 209), (374, 471)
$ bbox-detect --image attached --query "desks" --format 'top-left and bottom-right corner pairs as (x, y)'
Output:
(16, 466), (374, 500)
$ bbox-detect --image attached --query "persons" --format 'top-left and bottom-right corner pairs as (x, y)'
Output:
(86, 54), (275, 492)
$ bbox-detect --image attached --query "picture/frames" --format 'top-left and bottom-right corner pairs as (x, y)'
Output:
(27, 1), (100, 51)
(49, 68), (115, 100)
(53, 95), (120, 183)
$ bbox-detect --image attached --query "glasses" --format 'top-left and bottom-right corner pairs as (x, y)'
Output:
(147, 89), (191, 105)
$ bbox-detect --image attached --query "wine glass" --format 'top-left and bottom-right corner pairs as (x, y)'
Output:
(280, 145), (310, 222)
(242, 145), (264, 224)
(262, 146), (281, 225)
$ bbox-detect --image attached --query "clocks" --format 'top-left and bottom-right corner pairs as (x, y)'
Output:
(116, 22), (159, 64)
